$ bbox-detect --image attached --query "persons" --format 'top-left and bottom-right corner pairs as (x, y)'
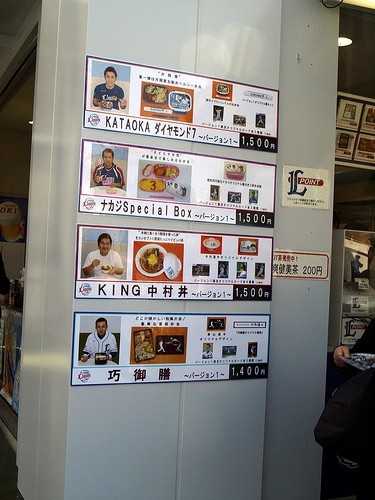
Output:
(348, 254), (363, 280)
(222, 344), (237, 358)
(230, 113), (265, 128)
(250, 191), (257, 203)
(208, 183), (243, 204)
(156, 339), (167, 354)
(82, 231), (124, 278)
(80, 316), (118, 367)
(215, 107), (223, 120)
(92, 148), (126, 190)
(166, 336), (183, 354)
(0, 244), (11, 313)
(201, 316), (226, 334)
(92, 65), (128, 113)
(310, 311), (374, 500)
(191, 259), (268, 281)
(247, 341), (259, 357)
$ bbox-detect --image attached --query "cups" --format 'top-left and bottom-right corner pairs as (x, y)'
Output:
(0, 202), (24, 243)
(202, 238), (220, 252)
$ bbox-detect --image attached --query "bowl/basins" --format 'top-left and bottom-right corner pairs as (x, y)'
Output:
(140, 178), (188, 199)
(94, 353), (107, 364)
(134, 329), (185, 363)
(100, 101), (113, 111)
(142, 162), (180, 180)
(216, 83), (230, 95)
(134, 243), (182, 280)
(100, 265), (112, 274)
(225, 165), (247, 181)
(142, 83), (169, 105)
